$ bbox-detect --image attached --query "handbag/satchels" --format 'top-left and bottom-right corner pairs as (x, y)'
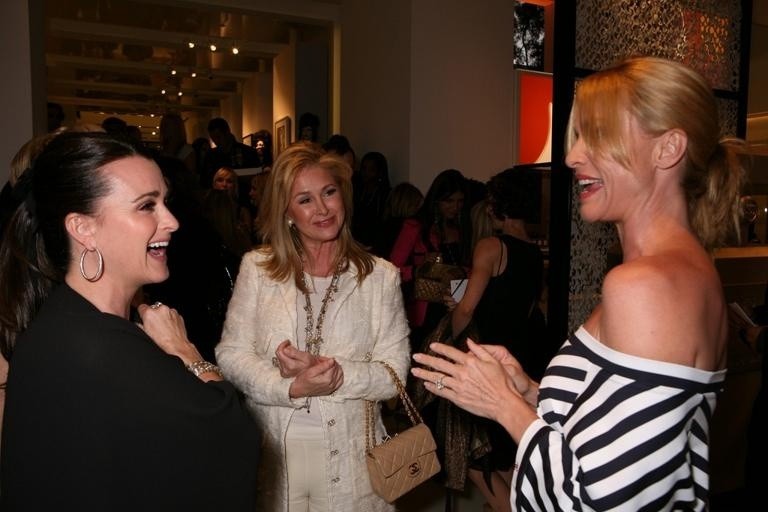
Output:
(415, 262), (466, 301)
(365, 360), (441, 505)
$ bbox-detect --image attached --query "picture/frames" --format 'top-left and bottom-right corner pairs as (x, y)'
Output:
(275, 117), (291, 158)
(242, 134), (254, 147)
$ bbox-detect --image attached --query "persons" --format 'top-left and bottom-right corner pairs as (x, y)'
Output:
(411, 56), (754, 512)
(2, 116), (547, 512)
